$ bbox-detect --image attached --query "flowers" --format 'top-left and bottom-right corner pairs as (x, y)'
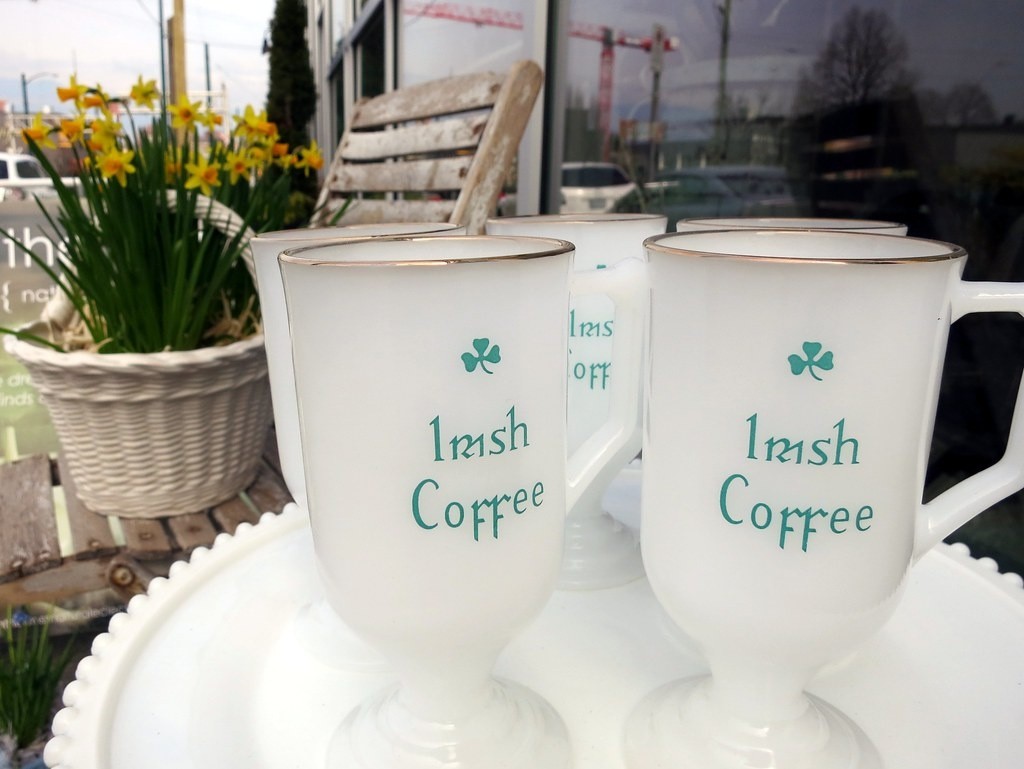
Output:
(0, 74), (363, 353)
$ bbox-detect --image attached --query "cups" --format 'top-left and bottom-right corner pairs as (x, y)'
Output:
(485, 213), (668, 593)
(646, 229), (1024, 769)
(248, 222), (466, 511)
(279, 237), (647, 769)
(675, 217), (907, 240)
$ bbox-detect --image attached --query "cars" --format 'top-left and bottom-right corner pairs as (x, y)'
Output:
(652, 167), (794, 216)
(0, 151), (47, 178)
(500, 158), (642, 215)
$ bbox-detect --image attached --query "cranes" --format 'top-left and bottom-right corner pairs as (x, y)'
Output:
(399, 1), (685, 160)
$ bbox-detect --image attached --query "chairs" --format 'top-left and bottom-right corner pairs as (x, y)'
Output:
(0, 60), (544, 609)
